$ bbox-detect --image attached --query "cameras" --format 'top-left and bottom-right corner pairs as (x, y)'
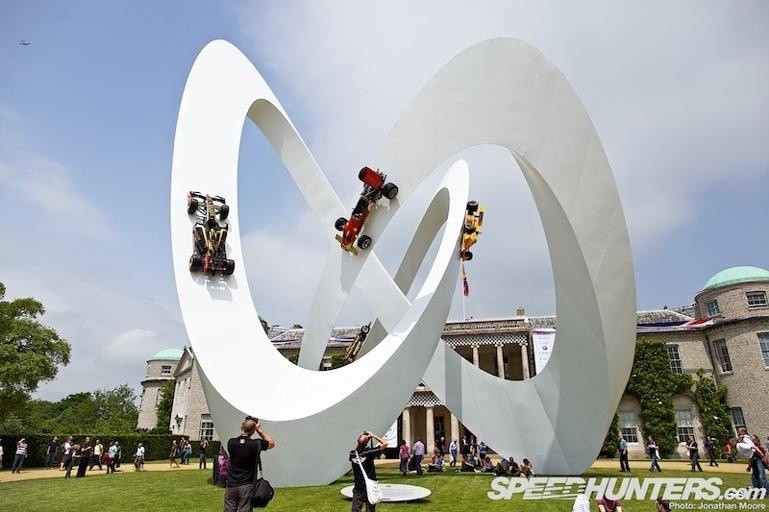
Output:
(245, 415), (259, 424)
(364, 433), (370, 443)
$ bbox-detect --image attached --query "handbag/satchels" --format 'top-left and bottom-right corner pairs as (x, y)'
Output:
(252, 477), (274, 508)
(366, 479), (383, 505)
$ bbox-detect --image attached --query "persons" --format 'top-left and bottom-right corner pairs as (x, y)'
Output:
(399, 440), (410, 476)
(223, 419), (275, 512)
(412, 436), (425, 475)
(686, 434), (703, 472)
(704, 427), (768, 499)
(597, 495), (623, 512)
(616, 436), (630, 472)
(349, 431), (388, 512)
(12, 438), (28, 474)
(656, 496), (673, 512)
(0, 439), (4, 481)
(647, 434), (662, 472)
(45, 435), (209, 479)
(427, 435), (536, 478)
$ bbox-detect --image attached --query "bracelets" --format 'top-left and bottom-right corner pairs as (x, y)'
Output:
(259, 431), (264, 435)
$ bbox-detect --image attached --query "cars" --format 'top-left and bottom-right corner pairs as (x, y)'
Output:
(178, 184), (236, 276)
